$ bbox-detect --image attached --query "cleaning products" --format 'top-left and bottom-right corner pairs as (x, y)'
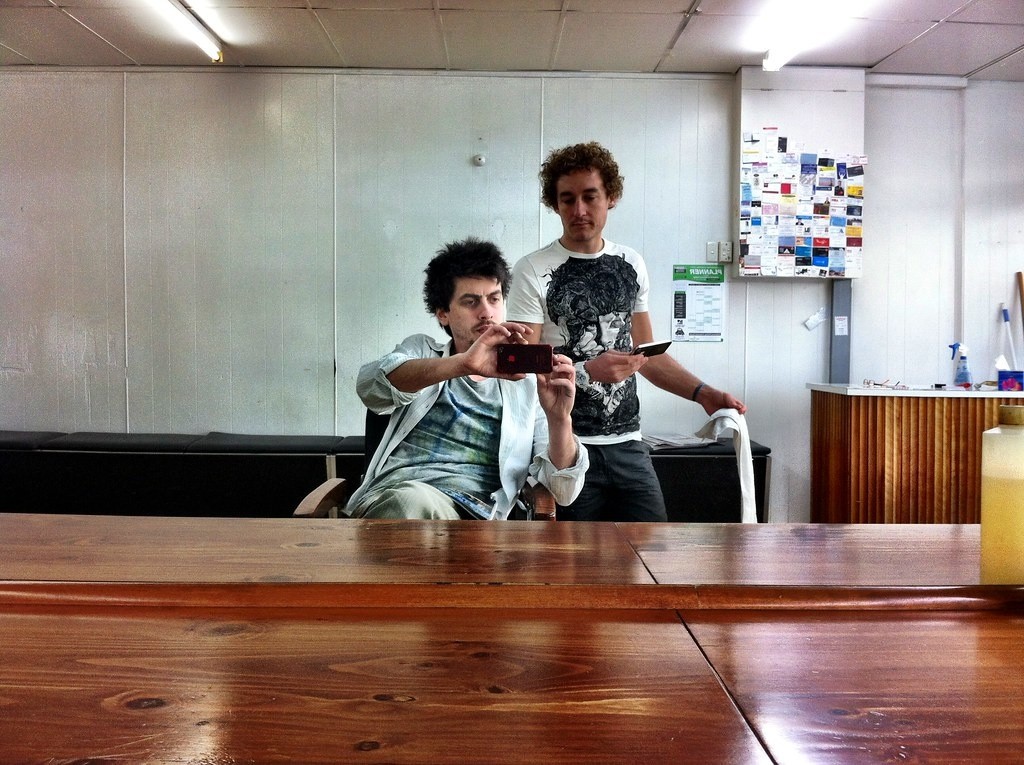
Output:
(949, 342), (973, 391)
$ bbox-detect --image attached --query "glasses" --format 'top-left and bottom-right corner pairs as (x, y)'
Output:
(863, 379), (900, 388)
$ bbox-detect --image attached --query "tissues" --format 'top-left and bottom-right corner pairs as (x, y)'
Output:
(995, 354), (1024, 391)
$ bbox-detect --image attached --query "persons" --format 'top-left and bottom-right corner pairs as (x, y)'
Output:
(343, 239), (591, 520)
(506, 142), (746, 522)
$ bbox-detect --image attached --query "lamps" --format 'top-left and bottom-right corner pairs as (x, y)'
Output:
(146, 0), (225, 66)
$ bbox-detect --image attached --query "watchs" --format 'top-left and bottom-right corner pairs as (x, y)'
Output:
(573, 361), (589, 387)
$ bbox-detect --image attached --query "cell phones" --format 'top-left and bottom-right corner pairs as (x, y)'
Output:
(496, 343), (553, 374)
(628, 341), (672, 357)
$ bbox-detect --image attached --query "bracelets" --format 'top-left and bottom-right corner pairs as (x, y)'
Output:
(692, 382), (705, 401)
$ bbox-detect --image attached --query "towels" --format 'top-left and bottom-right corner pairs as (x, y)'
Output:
(694, 409), (757, 523)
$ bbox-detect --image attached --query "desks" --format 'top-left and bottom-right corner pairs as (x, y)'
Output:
(805, 382), (1024, 525)
(0, 430), (64, 513)
(0, 509), (1024, 765)
(331, 436), (773, 523)
(37, 431), (343, 519)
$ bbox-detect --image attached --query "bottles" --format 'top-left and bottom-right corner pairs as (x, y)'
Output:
(979, 404), (1024, 585)
